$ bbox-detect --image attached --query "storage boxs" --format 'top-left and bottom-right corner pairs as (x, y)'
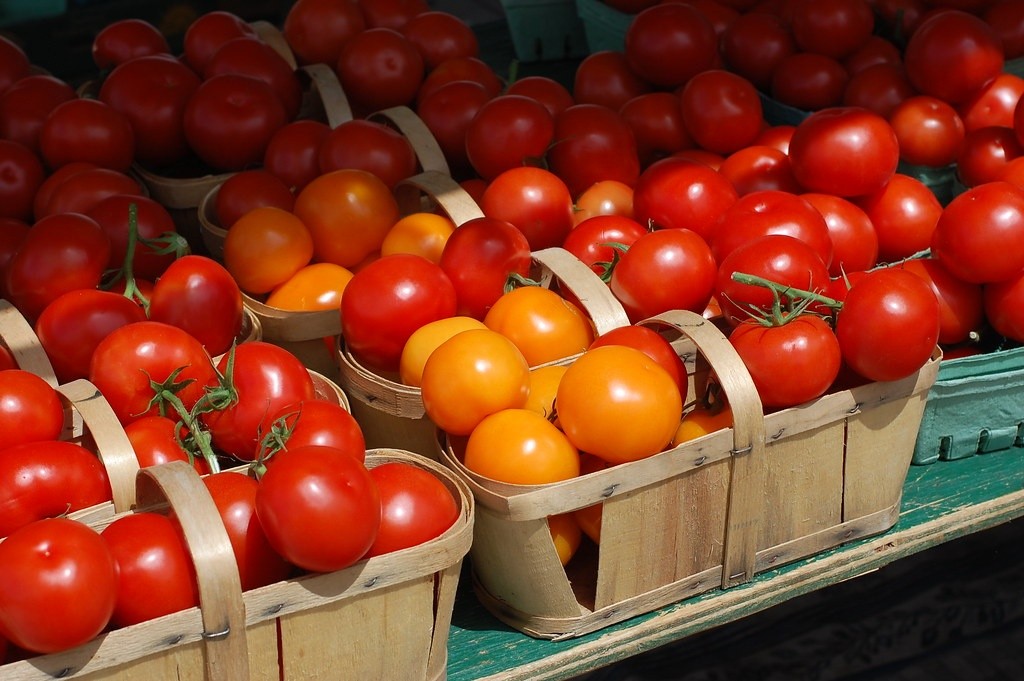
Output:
(501, 0), (1024, 464)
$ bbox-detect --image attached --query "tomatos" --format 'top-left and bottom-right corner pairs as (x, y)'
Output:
(0, 0), (1024, 661)
